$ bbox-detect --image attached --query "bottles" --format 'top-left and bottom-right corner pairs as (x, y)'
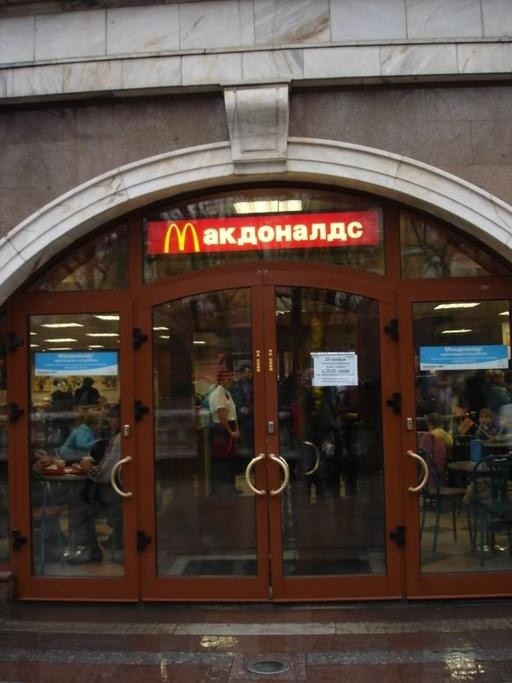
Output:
(469, 438), (482, 465)
(46, 456), (95, 470)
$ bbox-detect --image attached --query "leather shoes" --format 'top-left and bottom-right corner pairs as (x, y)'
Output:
(68, 545), (103, 565)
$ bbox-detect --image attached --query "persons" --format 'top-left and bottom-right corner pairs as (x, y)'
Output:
(158, 358), (359, 505)
(1, 377), (125, 567)
(414, 369), (511, 535)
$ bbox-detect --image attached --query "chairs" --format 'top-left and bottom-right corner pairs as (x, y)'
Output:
(415, 424), (512, 568)
(32, 480), (79, 575)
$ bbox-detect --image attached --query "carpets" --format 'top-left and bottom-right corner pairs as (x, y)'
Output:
(179, 556), (371, 574)
